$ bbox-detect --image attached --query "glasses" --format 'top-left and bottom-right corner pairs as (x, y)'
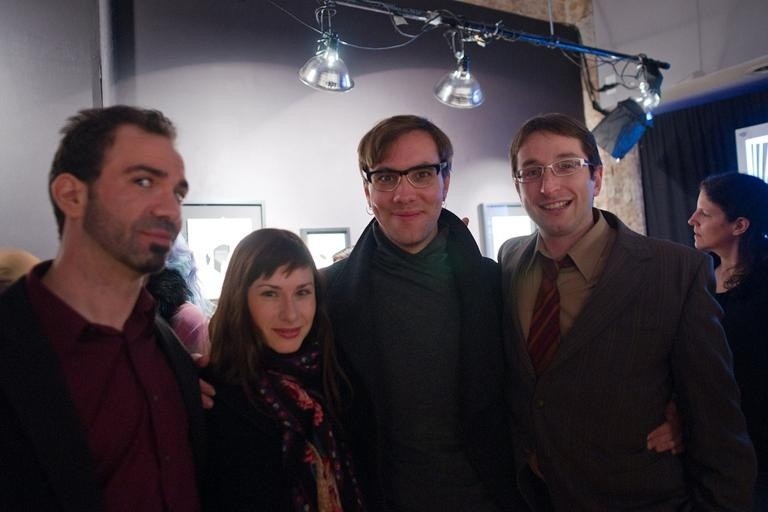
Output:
(360, 161), (448, 194)
(511, 157), (594, 184)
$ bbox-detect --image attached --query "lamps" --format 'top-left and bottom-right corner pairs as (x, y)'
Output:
(294, 0), (671, 164)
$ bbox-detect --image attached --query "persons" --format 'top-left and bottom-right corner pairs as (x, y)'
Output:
(498, 114), (757, 512)
(190, 116), (687, 512)
(1, 105), (204, 511)
(194, 227), (359, 511)
(0, 249), (40, 294)
(687, 173), (768, 476)
(156, 240), (208, 345)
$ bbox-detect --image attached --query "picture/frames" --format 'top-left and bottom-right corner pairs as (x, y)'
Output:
(479, 202), (539, 264)
(176, 201), (353, 310)
